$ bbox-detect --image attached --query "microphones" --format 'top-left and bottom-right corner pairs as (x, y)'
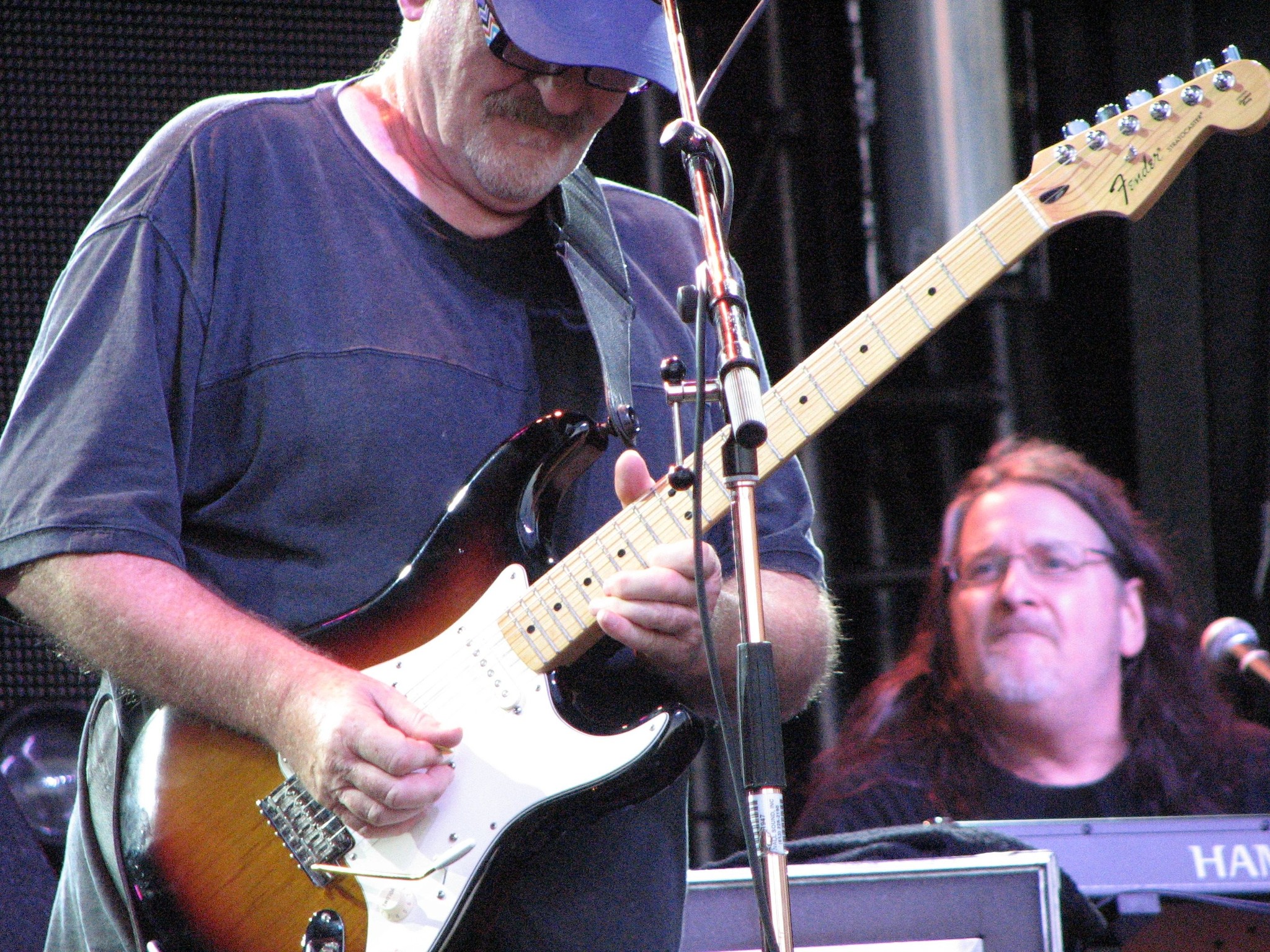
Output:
(1196, 614), (1268, 696)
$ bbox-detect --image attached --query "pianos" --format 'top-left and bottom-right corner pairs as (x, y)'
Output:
(913, 809), (1269, 900)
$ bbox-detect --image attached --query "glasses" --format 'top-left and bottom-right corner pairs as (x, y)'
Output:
(945, 541), (1125, 584)
(475, 0), (656, 96)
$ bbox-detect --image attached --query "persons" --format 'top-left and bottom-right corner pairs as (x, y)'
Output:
(784, 431), (1270, 952)
(0, 0), (841, 952)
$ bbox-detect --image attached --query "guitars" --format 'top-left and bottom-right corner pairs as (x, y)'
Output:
(73, 35), (1269, 952)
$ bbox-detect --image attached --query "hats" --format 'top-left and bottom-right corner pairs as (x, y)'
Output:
(490, 0), (687, 95)
